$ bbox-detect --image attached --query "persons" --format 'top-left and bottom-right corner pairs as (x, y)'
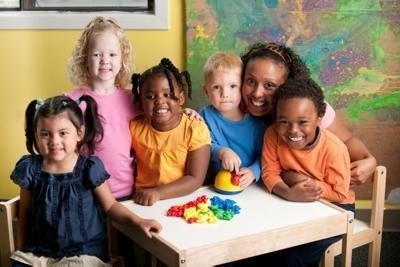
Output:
(9, 94), (163, 266)
(128, 57), (212, 205)
(199, 49), (266, 189)
(63, 15), (206, 206)
(241, 41), (378, 191)
(260, 75), (355, 266)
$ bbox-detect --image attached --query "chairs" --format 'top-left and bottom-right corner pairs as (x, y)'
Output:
(316, 162), (388, 267)
(1, 195), (125, 267)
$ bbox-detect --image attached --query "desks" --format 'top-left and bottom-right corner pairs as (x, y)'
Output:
(106, 179), (355, 267)
